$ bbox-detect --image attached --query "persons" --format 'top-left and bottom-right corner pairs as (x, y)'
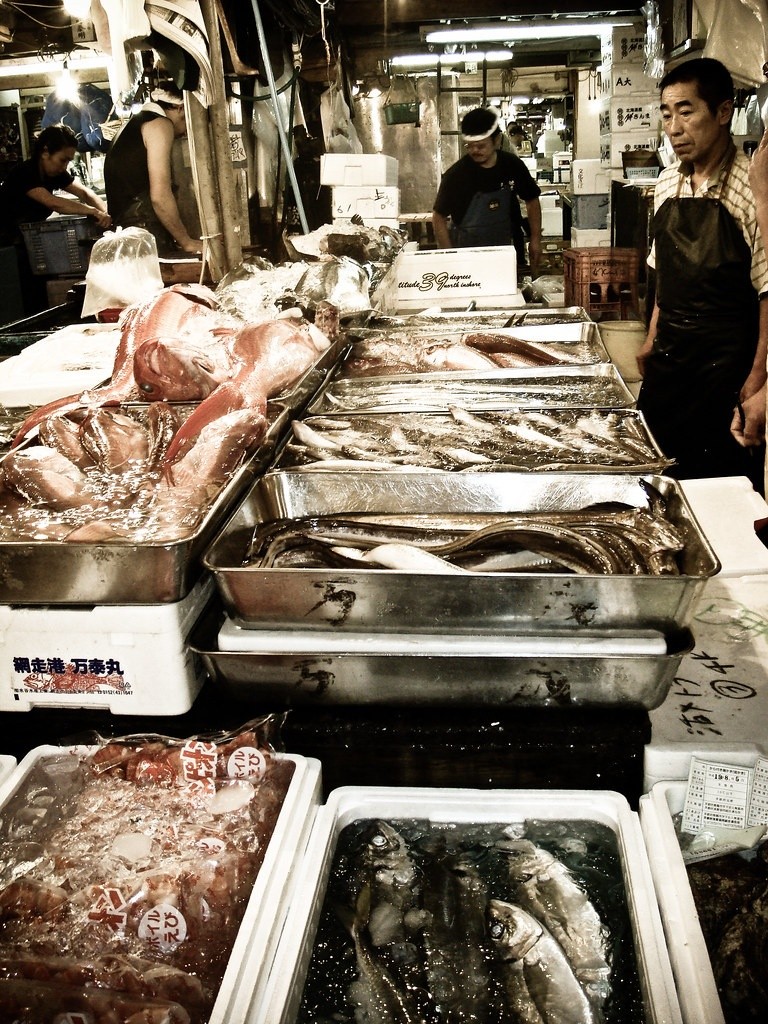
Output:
(506, 122), (518, 138)
(0, 125), (114, 328)
(104, 81), (206, 261)
(729, 130), (767, 449)
(510, 127), (539, 154)
(431, 108), (542, 284)
(293, 124), (330, 231)
(633, 57), (767, 497)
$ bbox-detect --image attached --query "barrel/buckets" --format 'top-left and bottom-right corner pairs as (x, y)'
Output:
(598, 320), (647, 382)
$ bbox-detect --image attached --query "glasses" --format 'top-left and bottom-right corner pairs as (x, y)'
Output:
(462, 137), (494, 149)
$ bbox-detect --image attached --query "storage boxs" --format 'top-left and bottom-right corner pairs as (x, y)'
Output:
(316, 151), (402, 188)
(1, 582), (216, 719)
(327, 187), (404, 219)
(565, 24), (666, 391)
(386, 246), (522, 303)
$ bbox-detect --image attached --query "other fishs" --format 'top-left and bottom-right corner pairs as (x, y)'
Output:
(693, 839), (768, 1024)
(0, 253), (688, 579)
(326, 817), (628, 1024)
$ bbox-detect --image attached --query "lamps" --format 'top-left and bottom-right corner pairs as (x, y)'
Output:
(388, 0), (645, 71)
